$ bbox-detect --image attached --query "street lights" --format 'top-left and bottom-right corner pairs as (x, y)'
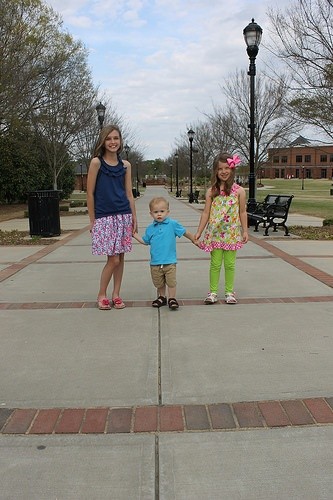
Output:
(186, 128), (196, 203)
(95, 101), (106, 131)
(123, 144), (130, 161)
(135, 159), (139, 197)
(301, 164), (306, 191)
(174, 152), (180, 197)
(169, 162), (173, 192)
(242, 17), (265, 225)
(79, 162), (84, 191)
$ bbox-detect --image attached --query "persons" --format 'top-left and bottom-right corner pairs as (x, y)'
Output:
(133, 197), (202, 308)
(86, 124), (138, 310)
(193, 152), (249, 304)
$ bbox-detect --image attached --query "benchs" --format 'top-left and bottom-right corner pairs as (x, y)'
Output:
(246, 194), (294, 237)
(187, 190), (200, 204)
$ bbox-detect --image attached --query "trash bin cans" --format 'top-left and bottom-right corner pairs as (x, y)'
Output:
(143, 183), (146, 187)
(26, 189), (64, 235)
(132, 189), (136, 198)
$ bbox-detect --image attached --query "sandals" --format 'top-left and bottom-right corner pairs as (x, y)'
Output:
(204, 293), (217, 303)
(97, 298), (112, 309)
(168, 298), (178, 308)
(112, 297), (125, 309)
(225, 293), (237, 303)
(151, 296), (167, 307)
